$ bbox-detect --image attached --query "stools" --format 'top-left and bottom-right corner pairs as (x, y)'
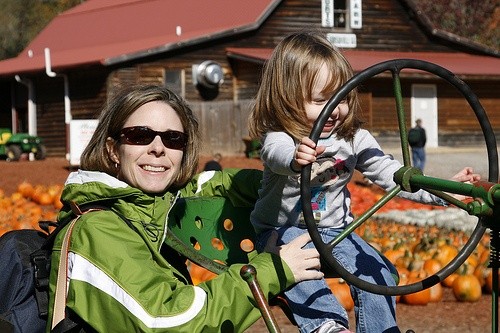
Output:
(163, 167), (399, 300)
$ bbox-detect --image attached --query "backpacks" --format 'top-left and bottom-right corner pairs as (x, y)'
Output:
(0, 228), (51, 333)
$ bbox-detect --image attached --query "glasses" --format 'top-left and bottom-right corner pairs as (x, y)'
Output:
(115, 126), (187, 153)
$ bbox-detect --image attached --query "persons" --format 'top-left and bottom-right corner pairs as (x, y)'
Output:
(407, 118), (427, 171)
(45, 82), (325, 333)
(246, 30), (481, 333)
